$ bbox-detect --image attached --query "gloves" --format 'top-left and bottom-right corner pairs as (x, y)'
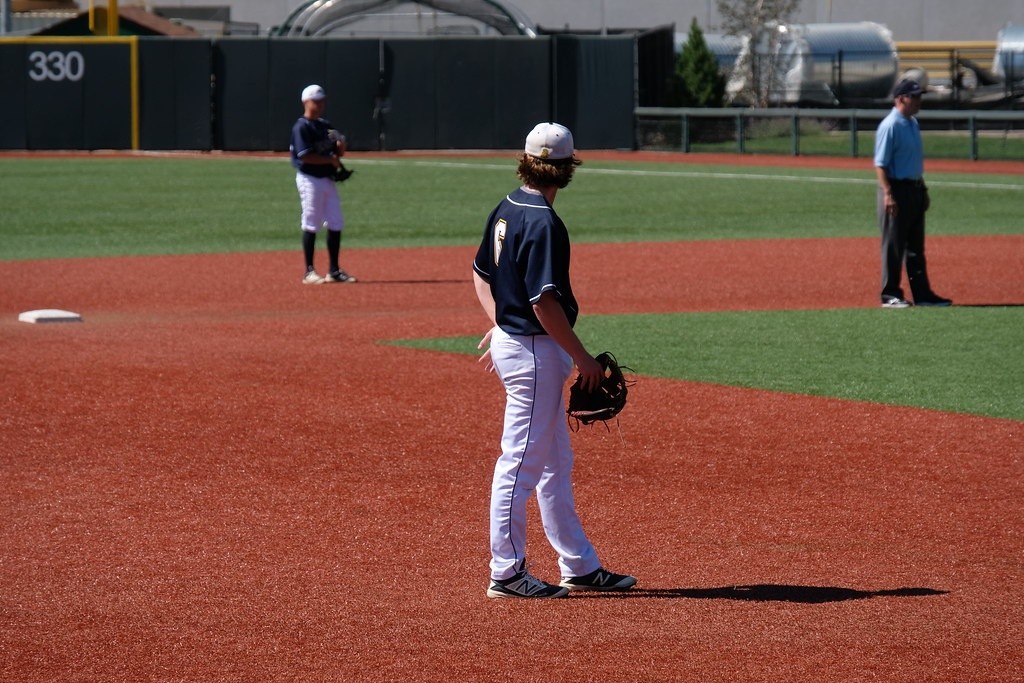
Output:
(327, 128), (346, 144)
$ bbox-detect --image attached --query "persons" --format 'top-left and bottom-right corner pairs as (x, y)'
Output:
(471, 119), (638, 599)
(289, 84), (358, 285)
(874, 78), (953, 309)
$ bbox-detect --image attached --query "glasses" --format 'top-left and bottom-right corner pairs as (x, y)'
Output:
(902, 93), (921, 100)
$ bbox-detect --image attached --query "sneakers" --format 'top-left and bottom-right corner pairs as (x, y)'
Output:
(487, 558), (570, 598)
(302, 265), (327, 284)
(325, 269), (357, 283)
(559, 566), (637, 592)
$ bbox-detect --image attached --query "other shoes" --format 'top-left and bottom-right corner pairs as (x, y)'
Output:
(881, 296), (912, 308)
(913, 294), (952, 306)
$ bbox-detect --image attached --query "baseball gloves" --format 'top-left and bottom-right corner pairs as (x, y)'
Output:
(330, 161), (354, 182)
(565, 352), (628, 426)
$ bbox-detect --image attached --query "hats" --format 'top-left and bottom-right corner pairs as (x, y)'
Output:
(893, 79), (928, 99)
(524, 122), (574, 160)
(301, 84), (326, 103)
